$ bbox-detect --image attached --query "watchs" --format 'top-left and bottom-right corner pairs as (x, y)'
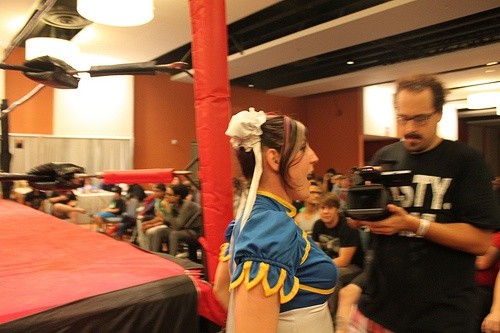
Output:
(413, 218), (430, 238)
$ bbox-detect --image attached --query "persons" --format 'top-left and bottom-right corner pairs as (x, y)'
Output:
(138, 181), (170, 253)
(310, 193), (367, 323)
(21, 183), (46, 215)
(305, 169), (348, 207)
(474, 231), (500, 333)
(47, 183), (87, 221)
(158, 184), (202, 257)
(93, 186), (125, 233)
(91, 170), (203, 257)
(332, 264), (370, 333)
(113, 183), (148, 241)
(347, 74), (491, 332)
(212, 110), (341, 333)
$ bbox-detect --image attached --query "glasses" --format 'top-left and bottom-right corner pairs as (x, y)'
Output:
(396, 109), (439, 124)
(165, 193), (176, 196)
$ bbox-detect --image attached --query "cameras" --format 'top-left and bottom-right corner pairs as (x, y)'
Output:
(344, 166), (413, 222)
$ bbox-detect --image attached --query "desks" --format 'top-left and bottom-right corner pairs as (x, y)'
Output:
(73, 189), (155, 225)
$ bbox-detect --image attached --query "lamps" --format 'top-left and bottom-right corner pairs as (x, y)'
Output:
(75, 0), (154, 27)
(25, 38), (80, 62)
